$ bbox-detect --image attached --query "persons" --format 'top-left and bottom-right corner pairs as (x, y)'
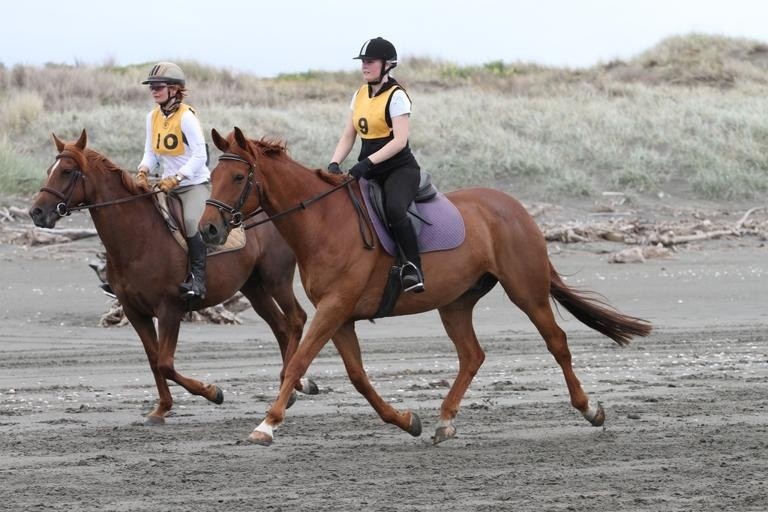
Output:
(93, 62), (210, 303)
(330, 37), (432, 296)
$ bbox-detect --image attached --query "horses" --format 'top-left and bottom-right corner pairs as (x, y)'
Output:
(197, 126), (654, 446)
(29, 127), (319, 427)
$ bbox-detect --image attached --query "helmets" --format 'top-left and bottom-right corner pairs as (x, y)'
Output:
(352, 37), (398, 67)
(141, 62), (186, 88)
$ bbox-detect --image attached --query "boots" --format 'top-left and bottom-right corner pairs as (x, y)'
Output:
(389, 218), (425, 295)
(99, 283), (117, 298)
(178, 232), (208, 300)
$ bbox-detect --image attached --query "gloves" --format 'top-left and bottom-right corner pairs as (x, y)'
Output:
(348, 157), (374, 179)
(158, 174), (179, 193)
(327, 161), (344, 175)
(135, 167), (148, 180)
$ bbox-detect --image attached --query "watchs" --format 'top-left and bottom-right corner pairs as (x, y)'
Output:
(175, 174), (183, 183)
